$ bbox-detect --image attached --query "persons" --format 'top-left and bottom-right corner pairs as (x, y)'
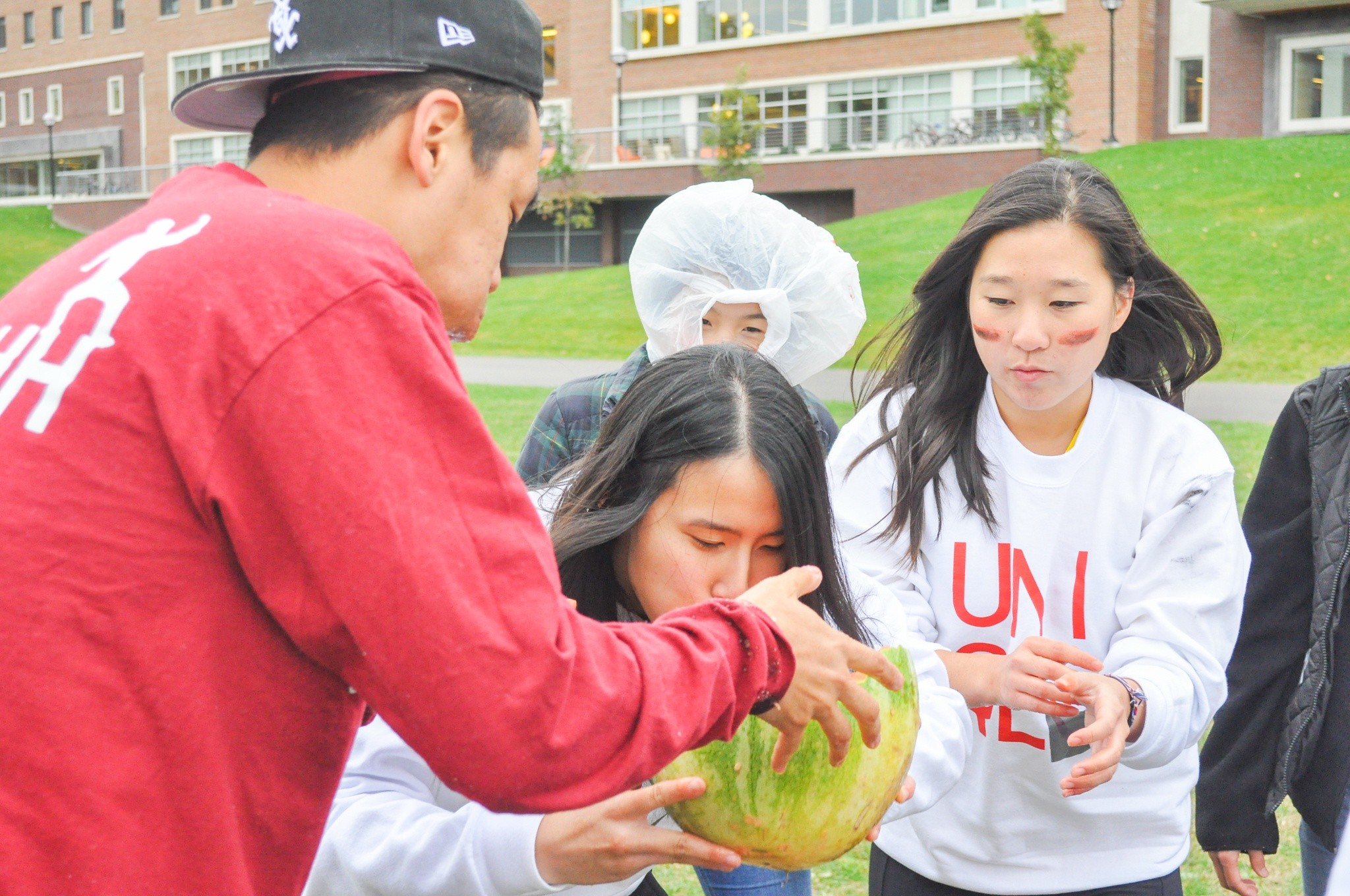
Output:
(516, 176), (869, 896)
(1195, 364), (1350, 896)
(0, 1), (901, 896)
(299, 339), (979, 896)
(826, 158), (1252, 896)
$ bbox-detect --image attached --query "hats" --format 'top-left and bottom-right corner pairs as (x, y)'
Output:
(169, 0), (546, 134)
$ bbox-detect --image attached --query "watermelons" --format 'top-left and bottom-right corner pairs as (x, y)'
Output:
(648, 645), (921, 869)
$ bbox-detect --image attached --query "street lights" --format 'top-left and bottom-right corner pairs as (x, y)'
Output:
(1101, 0), (1126, 144)
(609, 46), (628, 145)
(42, 112), (57, 197)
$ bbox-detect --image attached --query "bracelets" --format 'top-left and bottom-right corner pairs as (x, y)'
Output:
(1105, 672), (1146, 726)
